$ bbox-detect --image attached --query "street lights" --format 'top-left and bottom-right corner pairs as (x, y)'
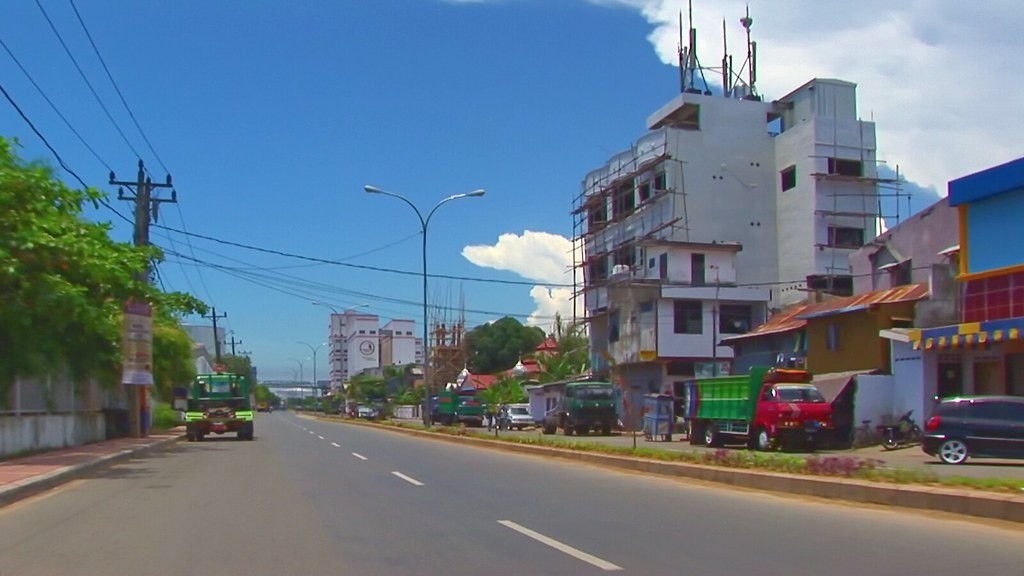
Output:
(296, 340), (329, 411)
(311, 300), (370, 417)
(363, 184), (485, 428)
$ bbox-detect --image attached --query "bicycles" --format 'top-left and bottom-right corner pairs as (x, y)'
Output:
(852, 414), (885, 449)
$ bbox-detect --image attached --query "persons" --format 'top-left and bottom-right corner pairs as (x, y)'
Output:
(485, 404), (507, 433)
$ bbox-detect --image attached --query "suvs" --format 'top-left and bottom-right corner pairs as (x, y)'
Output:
(920, 396), (1024, 465)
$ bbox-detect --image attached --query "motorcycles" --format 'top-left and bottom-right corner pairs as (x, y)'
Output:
(876, 408), (922, 451)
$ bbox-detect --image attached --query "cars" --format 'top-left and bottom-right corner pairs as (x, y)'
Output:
(495, 406), (536, 431)
(349, 401), (379, 420)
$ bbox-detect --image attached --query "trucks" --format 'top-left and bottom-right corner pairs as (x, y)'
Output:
(527, 375), (618, 436)
(171, 372), (256, 442)
(683, 365), (836, 451)
(437, 390), (487, 429)
